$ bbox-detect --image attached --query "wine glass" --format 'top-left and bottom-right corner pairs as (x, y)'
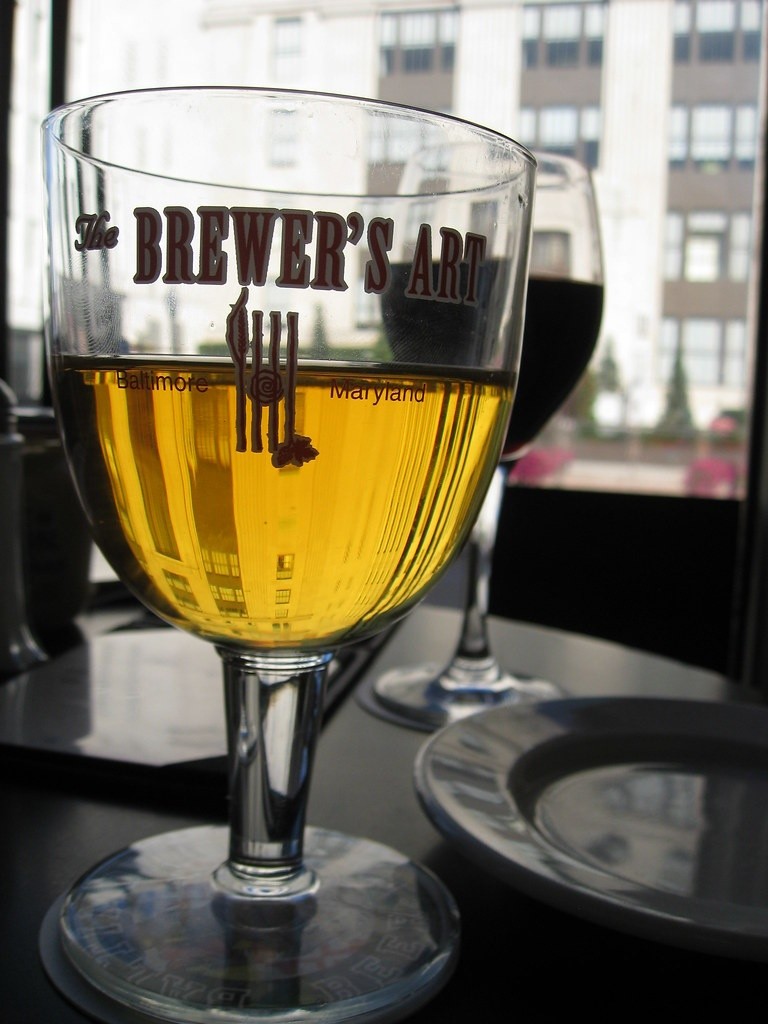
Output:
(34, 81), (537, 1024)
(370, 143), (604, 726)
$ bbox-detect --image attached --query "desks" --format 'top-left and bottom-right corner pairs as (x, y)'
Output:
(0, 607), (768, 1024)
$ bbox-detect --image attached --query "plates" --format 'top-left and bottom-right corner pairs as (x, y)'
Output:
(412, 694), (768, 964)
